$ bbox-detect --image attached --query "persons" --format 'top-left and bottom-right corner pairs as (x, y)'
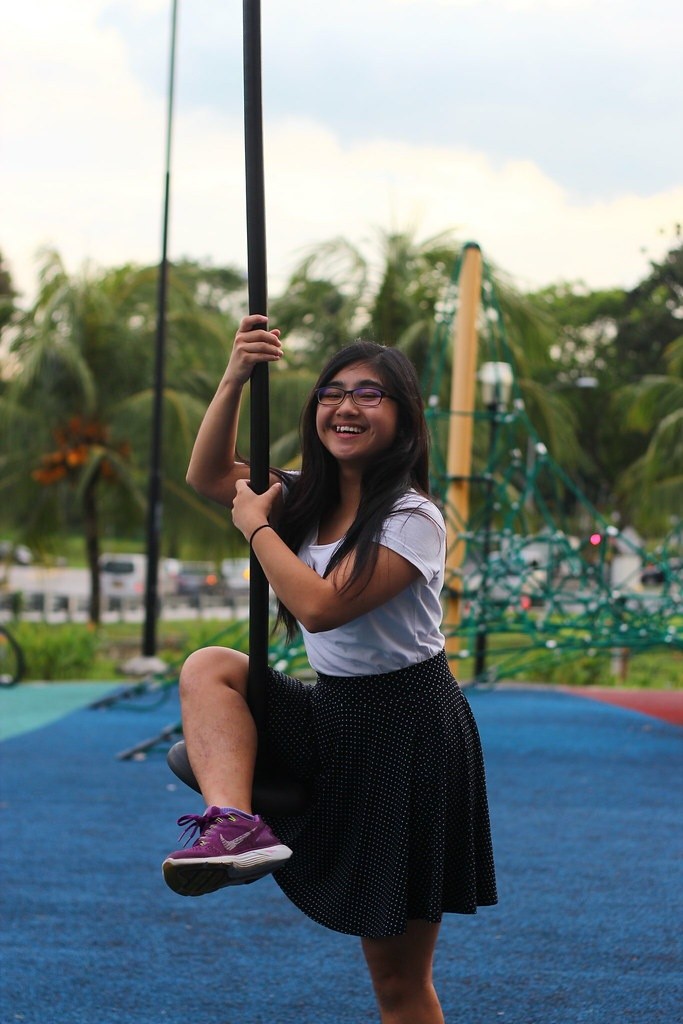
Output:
(521, 561), (546, 594)
(164, 314), (498, 1024)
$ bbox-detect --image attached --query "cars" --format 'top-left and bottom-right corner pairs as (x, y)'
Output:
(174, 563), (225, 594)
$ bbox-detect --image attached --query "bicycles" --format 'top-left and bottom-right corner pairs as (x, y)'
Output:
(0, 625), (26, 689)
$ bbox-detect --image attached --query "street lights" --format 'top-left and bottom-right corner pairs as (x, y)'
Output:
(473, 359), (515, 680)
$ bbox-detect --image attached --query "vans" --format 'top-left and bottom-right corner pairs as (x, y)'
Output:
(95, 551), (169, 609)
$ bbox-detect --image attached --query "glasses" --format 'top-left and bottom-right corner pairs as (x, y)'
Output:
(315, 386), (400, 406)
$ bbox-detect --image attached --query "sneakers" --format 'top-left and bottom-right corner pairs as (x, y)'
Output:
(162, 806), (293, 896)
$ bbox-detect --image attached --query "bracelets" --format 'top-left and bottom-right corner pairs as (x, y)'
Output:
(249, 525), (271, 546)
